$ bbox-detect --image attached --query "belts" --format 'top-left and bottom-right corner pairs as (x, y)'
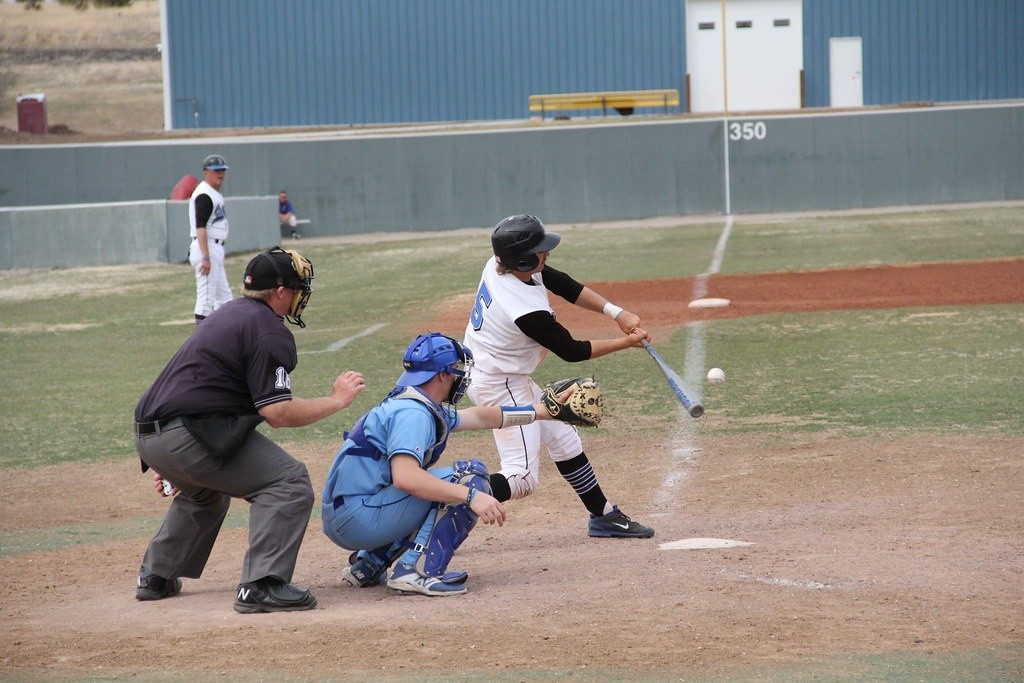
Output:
(214, 239), (225, 246)
(133, 416), (177, 434)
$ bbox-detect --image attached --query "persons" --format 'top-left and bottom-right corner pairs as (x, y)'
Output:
(188, 155), (233, 327)
(279, 190), (304, 240)
(323, 333), (604, 596)
(462, 215), (655, 538)
(134, 245), (366, 613)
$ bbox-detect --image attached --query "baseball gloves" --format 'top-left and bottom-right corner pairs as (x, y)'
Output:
(542, 376), (604, 427)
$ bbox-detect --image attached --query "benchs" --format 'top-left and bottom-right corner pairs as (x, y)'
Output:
(280, 218), (310, 225)
(529, 87), (680, 121)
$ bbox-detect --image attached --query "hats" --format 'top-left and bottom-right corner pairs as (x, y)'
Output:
(244, 247), (314, 292)
(202, 155), (229, 170)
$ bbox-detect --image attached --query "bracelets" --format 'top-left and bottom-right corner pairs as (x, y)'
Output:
(202, 256), (210, 261)
(462, 486), (478, 509)
(603, 302), (623, 320)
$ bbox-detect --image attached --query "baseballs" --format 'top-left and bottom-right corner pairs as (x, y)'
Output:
(707, 367), (725, 385)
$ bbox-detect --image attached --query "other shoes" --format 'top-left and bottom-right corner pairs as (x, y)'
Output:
(290, 232), (300, 240)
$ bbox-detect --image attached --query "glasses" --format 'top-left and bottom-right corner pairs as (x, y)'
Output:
(205, 168), (226, 172)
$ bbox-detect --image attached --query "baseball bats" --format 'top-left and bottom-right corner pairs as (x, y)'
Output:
(630, 328), (704, 418)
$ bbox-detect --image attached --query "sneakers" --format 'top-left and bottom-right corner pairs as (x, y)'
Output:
(342, 561), (388, 589)
(588, 505), (654, 540)
(135, 571), (182, 599)
(233, 578), (317, 613)
(386, 562), (467, 596)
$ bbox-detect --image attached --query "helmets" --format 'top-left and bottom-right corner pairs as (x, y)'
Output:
(397, 334), (463, 387)
(492, 215), (561, 272)
